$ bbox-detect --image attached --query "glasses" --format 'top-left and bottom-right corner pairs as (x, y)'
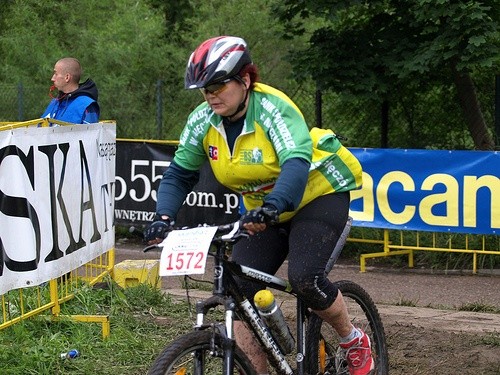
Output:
(199, 79), (233, 97)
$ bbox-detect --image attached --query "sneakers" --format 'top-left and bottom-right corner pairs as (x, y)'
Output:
(339, 328), (375, 375)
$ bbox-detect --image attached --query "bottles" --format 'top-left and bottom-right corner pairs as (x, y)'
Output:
(254, 290), (296, 356)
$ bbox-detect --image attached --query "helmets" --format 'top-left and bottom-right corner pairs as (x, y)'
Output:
(182, 35), (251, 90)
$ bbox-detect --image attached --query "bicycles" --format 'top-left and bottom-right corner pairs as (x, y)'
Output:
(144, 213), (389, 375)
